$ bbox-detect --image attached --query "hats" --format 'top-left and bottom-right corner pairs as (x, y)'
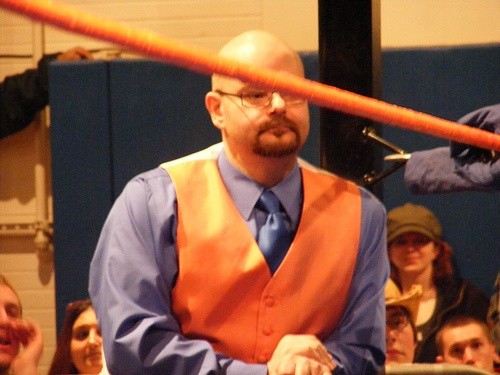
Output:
(386, 203), (442, 245)
(384, 278), (423, 323)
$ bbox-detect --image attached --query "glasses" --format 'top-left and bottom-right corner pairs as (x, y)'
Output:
(216, 86), (308, 109)
(385, 311), (413, 329)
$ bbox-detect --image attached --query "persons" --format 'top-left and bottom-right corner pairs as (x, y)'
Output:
(485, 288), (500, 361)
(47, 299), (105, 375)
(88, 29), (387, 375)
(386, 203), (490, 368)
(0, 274), (43, 375)
(385, 278), (423, 366)
(0, 47), (95, 137)
(435, 316), (496, 373)
